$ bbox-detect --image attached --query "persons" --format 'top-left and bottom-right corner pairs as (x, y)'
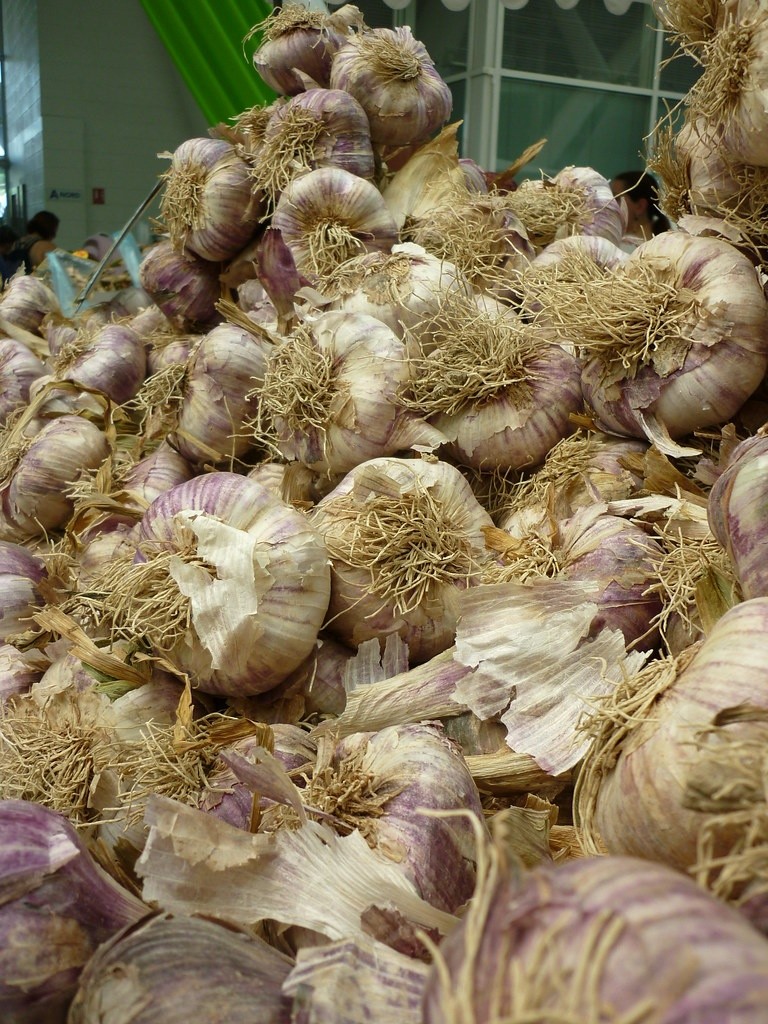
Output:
(609, 168), (670, 241)
(0, 211), (61, 291)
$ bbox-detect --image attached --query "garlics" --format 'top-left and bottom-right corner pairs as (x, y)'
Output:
(0, 0), (768, 1024)
